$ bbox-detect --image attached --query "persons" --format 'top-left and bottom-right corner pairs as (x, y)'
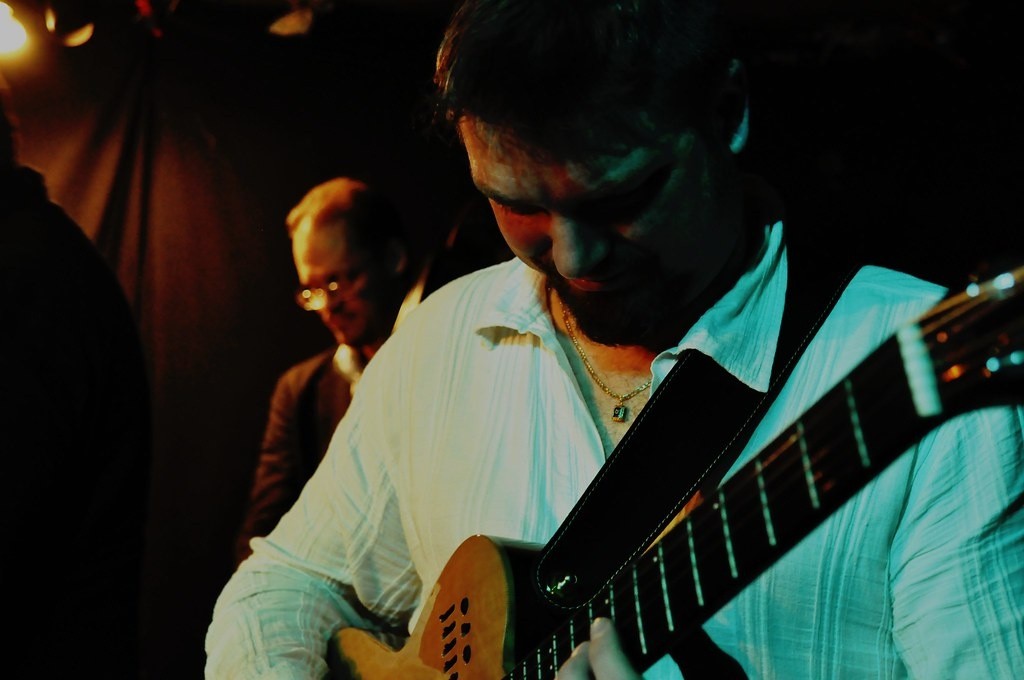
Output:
(206, 0), (1024, 680)
(239, 177), (408, 558)
(0, 70), (156, 680)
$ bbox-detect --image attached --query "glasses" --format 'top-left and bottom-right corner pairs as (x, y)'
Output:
(295, 251), (390, 314)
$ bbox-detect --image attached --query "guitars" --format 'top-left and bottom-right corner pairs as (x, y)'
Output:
(324, 266), (1023, 680)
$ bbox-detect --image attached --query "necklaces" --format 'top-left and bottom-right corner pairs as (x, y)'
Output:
(559, 301), (651, 423)
